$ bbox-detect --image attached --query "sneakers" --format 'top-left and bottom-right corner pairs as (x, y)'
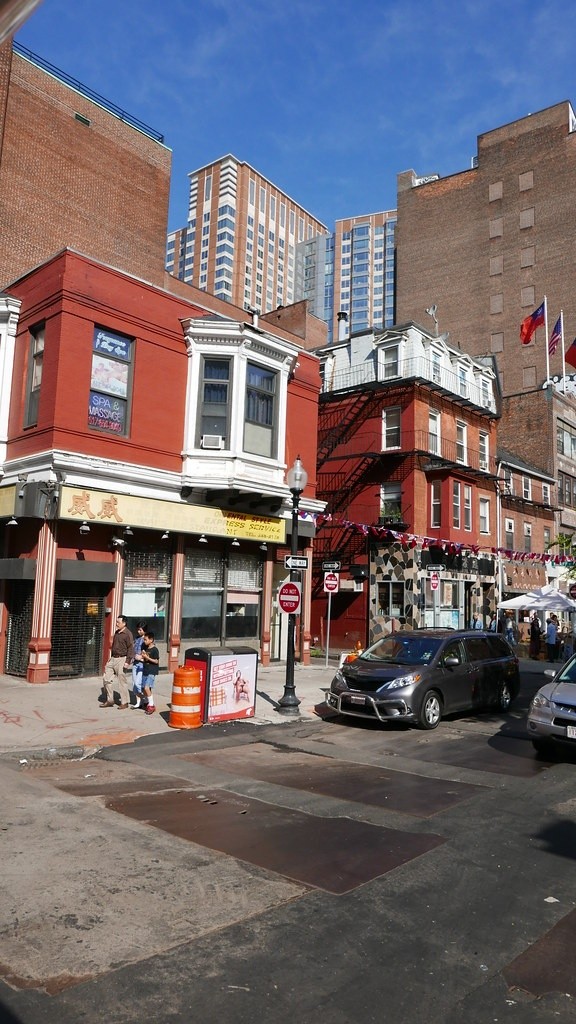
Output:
(145, 705), (155, 715)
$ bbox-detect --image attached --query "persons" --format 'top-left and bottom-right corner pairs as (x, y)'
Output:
(530, 612), (559, 662)
(100, 615), (159, 714)
(470, 609), (516, 646)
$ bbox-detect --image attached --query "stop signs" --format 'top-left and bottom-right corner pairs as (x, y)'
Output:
(323, 571), (340, 592)
(430, 573), (440, 591)
(278, 581), (303, 615)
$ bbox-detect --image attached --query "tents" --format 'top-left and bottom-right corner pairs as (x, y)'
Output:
(496, 584), (576, 633)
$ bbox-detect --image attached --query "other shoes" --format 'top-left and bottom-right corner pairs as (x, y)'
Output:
(130, 705), (142, 709)
(143, 702), (148, 707)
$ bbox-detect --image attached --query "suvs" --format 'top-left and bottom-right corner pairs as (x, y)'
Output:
(323, 625), (521, 730)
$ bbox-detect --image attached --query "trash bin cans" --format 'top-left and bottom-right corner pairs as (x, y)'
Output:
(184, 645), (258, 723)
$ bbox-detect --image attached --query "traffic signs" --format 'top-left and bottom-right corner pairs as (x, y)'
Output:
(284, 555), (308, 571)
(322, 561), (341, 571)
(425, 565), (447, 572)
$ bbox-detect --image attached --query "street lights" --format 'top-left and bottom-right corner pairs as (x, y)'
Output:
(270, 453), (307, 716)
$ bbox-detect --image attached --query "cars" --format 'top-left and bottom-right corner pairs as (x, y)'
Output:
(526, 652), (576, 751)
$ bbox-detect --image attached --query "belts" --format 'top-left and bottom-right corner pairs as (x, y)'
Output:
(113, 655), (126, 657)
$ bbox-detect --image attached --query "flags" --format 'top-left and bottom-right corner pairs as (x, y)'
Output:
(565, 337), (576, 369)
(520, 301), (544, 344)
(548, 316), (561, 354)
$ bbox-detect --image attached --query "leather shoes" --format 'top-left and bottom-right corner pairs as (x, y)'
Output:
(118, 703), (128, 709)
(100, 701), (113, 707)
